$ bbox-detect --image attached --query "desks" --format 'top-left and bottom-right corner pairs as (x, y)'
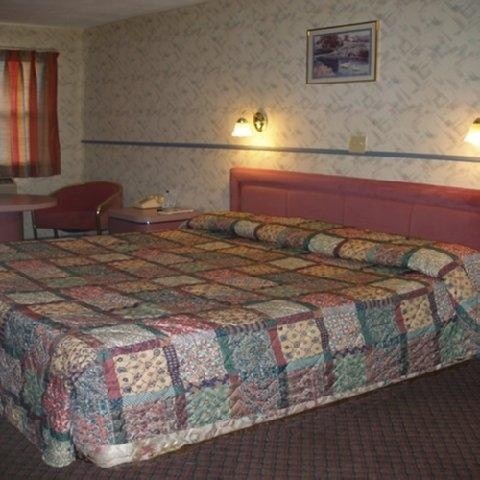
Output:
(0, 193), (58, 242)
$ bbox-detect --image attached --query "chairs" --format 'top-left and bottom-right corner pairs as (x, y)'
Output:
(30, 180), (124, 238)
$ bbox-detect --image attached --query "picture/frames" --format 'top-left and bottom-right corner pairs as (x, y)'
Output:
(305, 19), (381, 85)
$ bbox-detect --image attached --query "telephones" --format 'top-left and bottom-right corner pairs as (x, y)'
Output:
(132, 196), (164, 209)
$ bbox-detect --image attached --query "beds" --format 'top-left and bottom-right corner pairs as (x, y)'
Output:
(0, 167), (480, 470)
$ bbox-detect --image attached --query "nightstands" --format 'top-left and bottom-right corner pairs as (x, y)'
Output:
(104, 207), (202, 233)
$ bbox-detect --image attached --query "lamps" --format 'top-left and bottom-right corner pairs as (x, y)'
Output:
(229, 109), (268, 138)
(462, 117), (480, 147)
